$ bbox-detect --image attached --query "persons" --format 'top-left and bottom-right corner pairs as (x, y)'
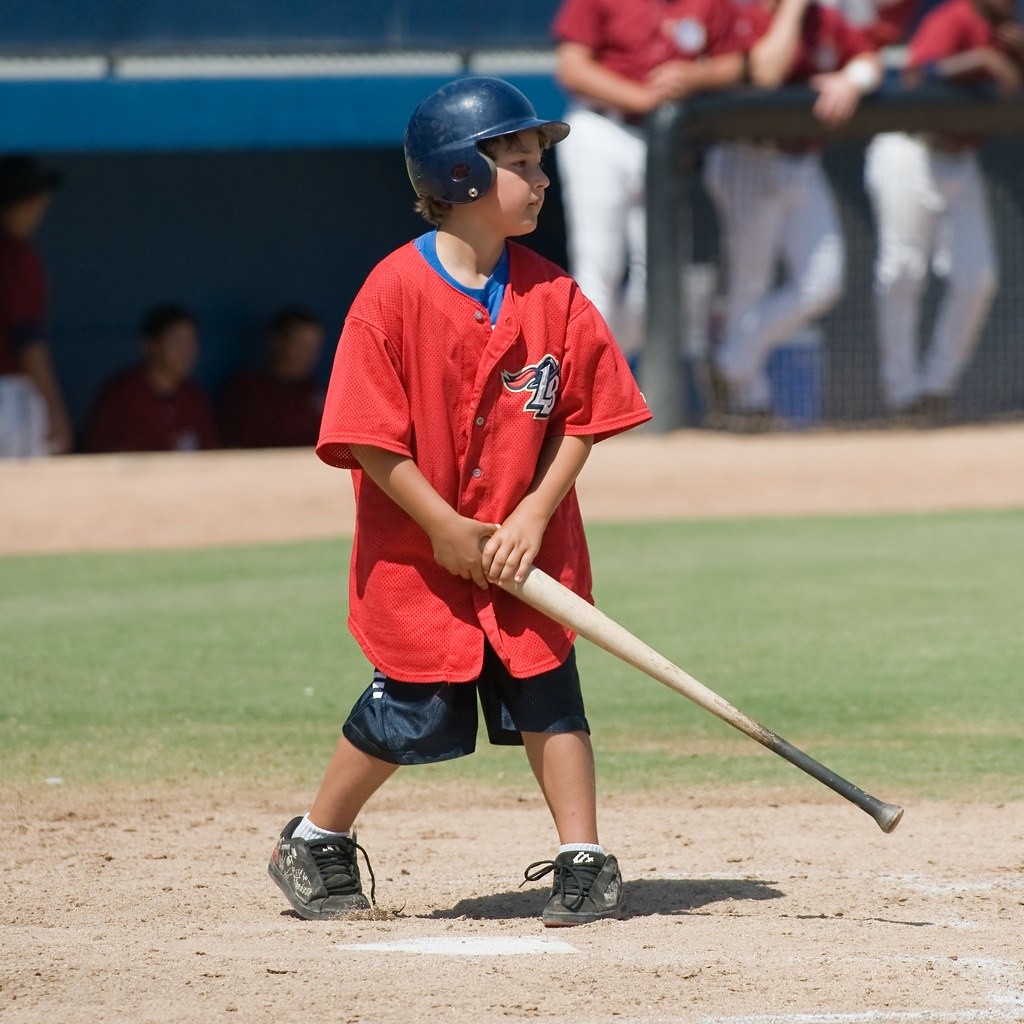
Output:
(0, 151), (77, 458)
(267, 76), (653, 921)
(83, 304), (216, 451)
(551, 0), (1024, 431)
(219, 308), (327, 447)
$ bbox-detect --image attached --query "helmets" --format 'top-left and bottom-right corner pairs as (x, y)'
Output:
(404, 75), (570, 204)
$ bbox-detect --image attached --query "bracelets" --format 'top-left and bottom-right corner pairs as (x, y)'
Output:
(847, 60), (880, 90)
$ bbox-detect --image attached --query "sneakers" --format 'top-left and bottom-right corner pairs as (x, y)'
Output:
(266, 815), (376, 920)
(519, 849), (630, 927)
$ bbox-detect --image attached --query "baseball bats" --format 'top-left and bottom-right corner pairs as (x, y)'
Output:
(477, 535), (904, 834)
